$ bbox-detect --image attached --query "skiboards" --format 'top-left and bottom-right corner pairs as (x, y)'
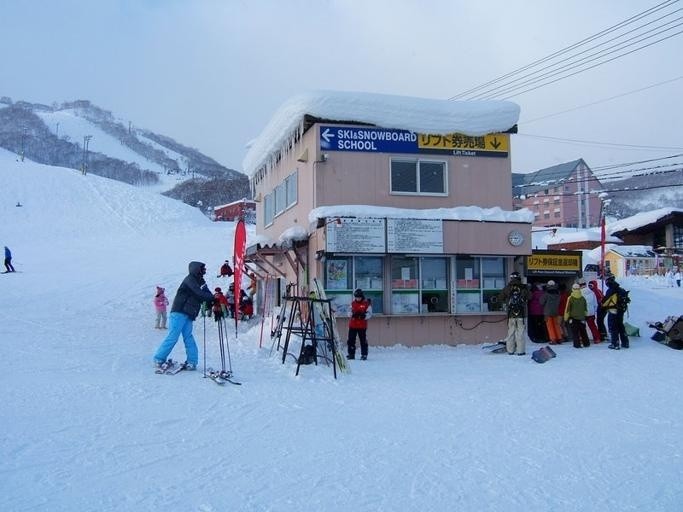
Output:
(154, 358), (186, 374)
(267, 278), (348, 374)
(203, 368), (242, 385)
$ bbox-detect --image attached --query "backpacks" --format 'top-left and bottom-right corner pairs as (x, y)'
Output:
(617, 289), (629, 313)
(508, 287), (524, 310)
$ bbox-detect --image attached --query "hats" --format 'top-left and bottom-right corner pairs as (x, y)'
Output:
(579, 278), (586, 284)
(157, 287), (164, 293)
(215, 288), (220, 291)
(571, 284), (579, 291)
(354, 289), (363, 297)
(225, 260), (229, 263)
(548, 280), (555, 286)
(510, 272), (520, 280)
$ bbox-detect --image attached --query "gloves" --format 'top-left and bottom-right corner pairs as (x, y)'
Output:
(352, 313), (365, 319)
(213, 301), (224, 321)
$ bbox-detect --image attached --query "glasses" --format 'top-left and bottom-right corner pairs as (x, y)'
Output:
(588, 284), (594, 287)
(160, 288), (165, 292)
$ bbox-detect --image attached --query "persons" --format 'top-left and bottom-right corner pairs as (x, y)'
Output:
(499, 271), (632, 356)
(346, 288), (373, 360)
(153, 260), (256, 371)
(4, 245), (15, 273)
(664, 269), (681, 287)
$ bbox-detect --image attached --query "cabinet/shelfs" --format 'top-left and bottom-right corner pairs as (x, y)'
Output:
(277, 296), (336, 379)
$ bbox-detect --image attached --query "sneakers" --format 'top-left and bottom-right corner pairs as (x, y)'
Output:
(565, 336), (608, 348)
(155, 361), (163, 371)
(621, 344), (629, 348)
(187, 363), (194, 370)
(509, 352), (514, 355)
(549, 340), (560, 345)
(162, 327), (168, 329)
(346, 354), (355, 359)
(155, 327), (161, 329)
(518, 352), (525, 355)
(608, 344), (619, 349)
(361, 355), (367, 360)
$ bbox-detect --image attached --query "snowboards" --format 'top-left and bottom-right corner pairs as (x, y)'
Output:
(532, 346), (556, 364)
(482, 343), (507, 351)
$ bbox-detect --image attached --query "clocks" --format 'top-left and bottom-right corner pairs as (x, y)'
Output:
(508, 230), (524, 246)
